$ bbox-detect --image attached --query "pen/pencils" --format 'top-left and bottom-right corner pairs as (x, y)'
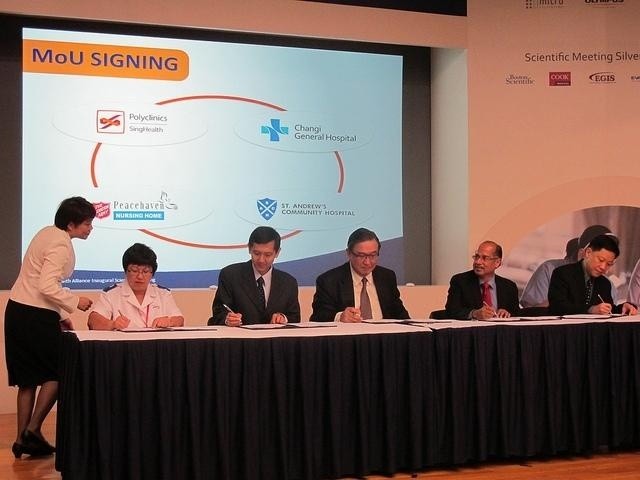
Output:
(353, 312), (355, 316)
(597, 294), (612, 314)
(223, 304), (242, 322)
(117, 310), (129, 329)
(483, 301), (497, 315)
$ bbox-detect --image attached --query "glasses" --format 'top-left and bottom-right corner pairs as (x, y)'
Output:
(351, 251), (379, 260)
(472, 253), (498, 262)
(126, 266), (153, 276)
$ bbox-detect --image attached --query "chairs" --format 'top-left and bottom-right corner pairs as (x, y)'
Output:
(430, 307), (551, 317)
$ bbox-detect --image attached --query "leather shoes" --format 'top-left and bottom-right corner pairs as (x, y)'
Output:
(12, 429), (56, 458)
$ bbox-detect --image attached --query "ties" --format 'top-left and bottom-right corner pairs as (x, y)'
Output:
(360, 278), (372, 320)
(256, 276), (265, 313)
(585, 278), (593, 305)
(483, 282), (492, 307)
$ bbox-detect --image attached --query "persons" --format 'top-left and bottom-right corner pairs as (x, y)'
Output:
(4, 197), (96, 459)
(548, 233), (637, 316)
(88, 244), (184, 329)
(519, 225), (617, 308)
(208, 226), (300, 326)
(445, 241), (517, 318)
(310, 228), (410, 320)
